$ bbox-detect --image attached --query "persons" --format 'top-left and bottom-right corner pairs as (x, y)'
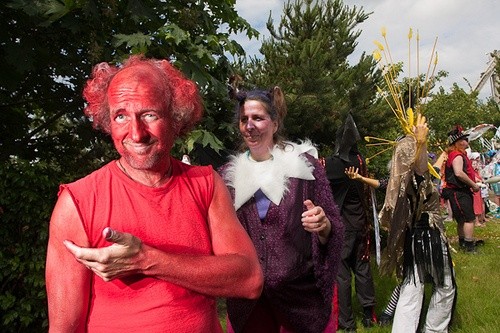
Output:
(45, 54), (263, 333)
(216, 85), (345, 333)
(326, 114), (500, 333)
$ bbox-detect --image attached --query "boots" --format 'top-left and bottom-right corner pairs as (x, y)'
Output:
(362, 306), (381, 328)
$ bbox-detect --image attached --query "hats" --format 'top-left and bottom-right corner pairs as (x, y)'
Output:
(488, 150), (496, 157)
(447, 130), (471, 147)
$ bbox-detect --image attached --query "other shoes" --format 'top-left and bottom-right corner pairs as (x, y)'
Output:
(444, 218), (453, 224)
(463, 240), (484, 249)
(458, 238), (466, 248)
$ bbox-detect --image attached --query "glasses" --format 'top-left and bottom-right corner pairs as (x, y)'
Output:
(461, 137), (469, 141)
